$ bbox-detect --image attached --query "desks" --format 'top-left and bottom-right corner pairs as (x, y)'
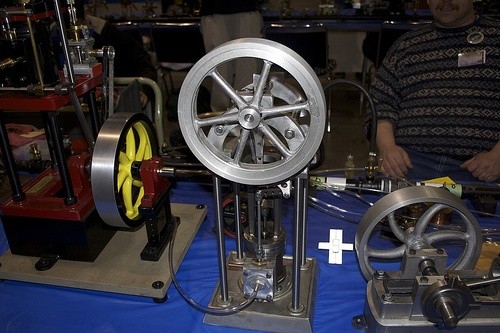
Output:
(112, 9), (431, 76)
(0, 170), (500, 333)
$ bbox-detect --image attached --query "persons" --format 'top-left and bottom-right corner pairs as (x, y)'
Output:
(361, 0), (500, 185)
(83, 6), (157, 109)
(196, 0), (264, 114)
